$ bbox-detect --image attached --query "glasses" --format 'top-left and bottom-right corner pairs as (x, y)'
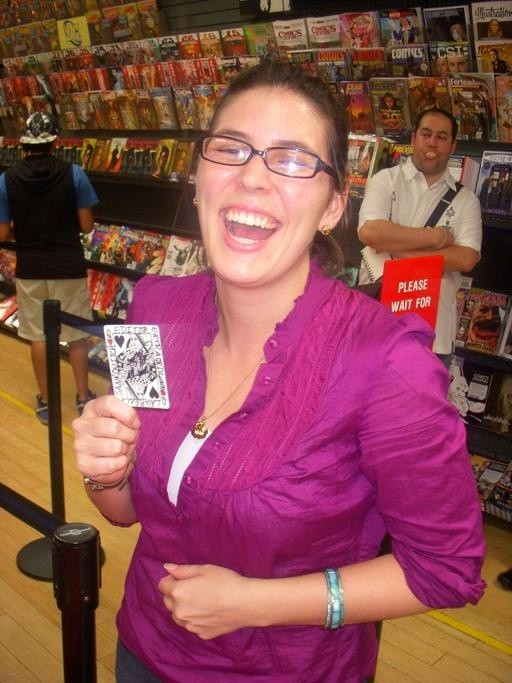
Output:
(196, 135), (342, 183)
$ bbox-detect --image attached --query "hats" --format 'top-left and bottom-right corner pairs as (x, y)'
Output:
(20, 111), (61, 145)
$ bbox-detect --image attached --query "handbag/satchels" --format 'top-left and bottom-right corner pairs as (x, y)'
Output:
(348, 181), (465, 305)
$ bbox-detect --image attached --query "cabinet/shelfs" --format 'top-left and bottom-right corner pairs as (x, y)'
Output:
(4, 6), (510, 531)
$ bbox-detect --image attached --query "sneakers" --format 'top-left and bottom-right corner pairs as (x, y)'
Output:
(34, 393), (50, 425)
(76, 390), (96, 416)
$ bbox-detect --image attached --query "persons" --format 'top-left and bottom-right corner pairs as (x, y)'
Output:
(356, 106), (483, 374)
(0, 110), (99, 426)
(70, 61), (486, 683)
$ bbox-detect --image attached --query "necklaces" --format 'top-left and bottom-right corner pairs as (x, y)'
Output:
(191, 343), (282, 440)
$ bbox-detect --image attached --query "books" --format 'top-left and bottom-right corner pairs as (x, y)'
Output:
(239, 57), (260, 80)
(485, 369), (512, 441)
(244, 23), (281, 56)
(336, 262), (358, 286)
(478, 150), (512, 217)
(1, 141), (25, 171)
(451, 289), (512, 355)
(370, 75), (414, 142)
(493, 78), (511, 145)
(287, 50), (316, 81)
(272, 18), (309, 62)
(428, 39), (472, 76)
(346, 44), (387, 83)
(445, 360), (491, 430)
(472, 0), (512, 41)
(475, 38), (511, 74)
(346, 135), (391, 185)
(408, 74), (453, 129)
(337, 80), (376, 135)
(318, 48), (350, 82)
(53, 137), (192, 180)
(305, 12), (342, 53)
(0, 244), (20, 336)
(395, 143), (482, 196)
(452, 273), (474, 344)
(218, 57), (239, 84)
(378, 4), (425, 48)
(0, 1), (216, 134)
(341, 9), (384, 49)
(470, 455), (511, 524)
(81, 217), (212, 372)
(450, 70), (499, 142)
(424, 2), (473, 71)
(221, 29), (247, 58)
(360, 245), (396, 283)
(392, 42), (432, 77)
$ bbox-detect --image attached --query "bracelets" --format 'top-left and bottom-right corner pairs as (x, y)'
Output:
(323, 567), (345, 629)
(436, 225), (447, 251)
(83, 449), (139, 491)
